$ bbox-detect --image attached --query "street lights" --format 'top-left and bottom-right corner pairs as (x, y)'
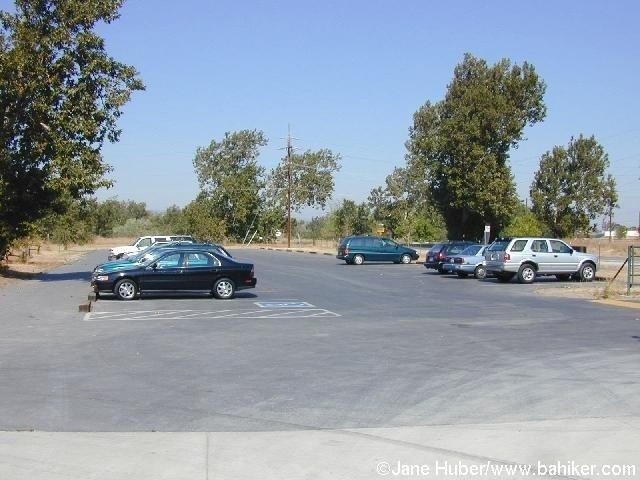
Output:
(520, 197), (528, 213)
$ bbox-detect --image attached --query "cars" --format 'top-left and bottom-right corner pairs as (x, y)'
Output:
(443, 244), (497, 279)
(90, 231), (258, 302)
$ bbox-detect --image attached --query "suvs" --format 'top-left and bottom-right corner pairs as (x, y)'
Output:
(485, 236), (601, 285)
(424, 240), (480, 273)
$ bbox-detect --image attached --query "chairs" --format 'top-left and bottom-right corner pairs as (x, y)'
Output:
(540, 243), (546, 252)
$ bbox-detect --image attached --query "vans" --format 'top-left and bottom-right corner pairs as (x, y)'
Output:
(335, 235), (422, 266)
